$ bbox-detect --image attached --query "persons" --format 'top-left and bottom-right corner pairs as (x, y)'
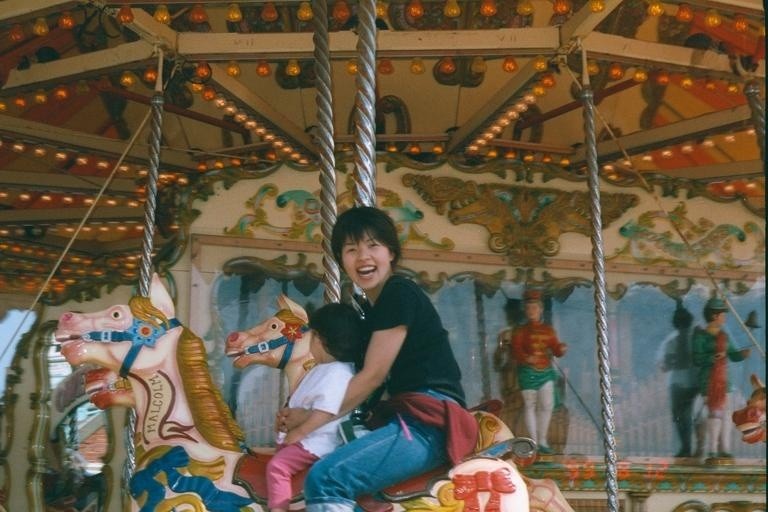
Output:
(495, 299), (522, 437)
(657, 307), (706, 458)
(510, 287), (568, 455)
(691, 289), (751, 457)
(264, 301), (362, 512)
(273, 205), (469, 512)
